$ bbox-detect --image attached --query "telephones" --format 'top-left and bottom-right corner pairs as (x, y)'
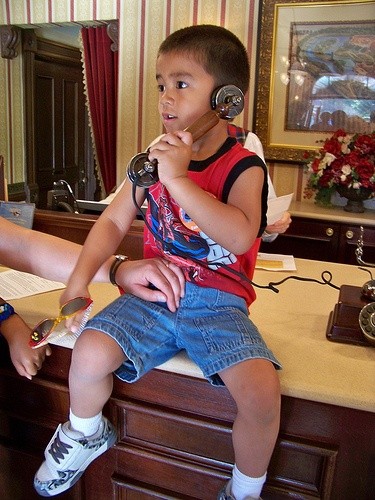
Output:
(125, 84), (245, 189)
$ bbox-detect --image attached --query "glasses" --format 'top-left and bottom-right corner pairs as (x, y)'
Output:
(28, 297), (93, 348)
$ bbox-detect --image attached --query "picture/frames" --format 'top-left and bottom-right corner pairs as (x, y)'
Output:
(252, 0), (375, 164)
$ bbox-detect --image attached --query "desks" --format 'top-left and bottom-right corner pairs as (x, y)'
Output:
(1, 258), (375, 499)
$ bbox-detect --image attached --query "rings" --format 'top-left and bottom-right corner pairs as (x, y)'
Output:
(166, 261), (172, 267)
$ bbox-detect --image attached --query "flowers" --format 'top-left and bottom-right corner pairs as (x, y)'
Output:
(301, 128), (375, 209)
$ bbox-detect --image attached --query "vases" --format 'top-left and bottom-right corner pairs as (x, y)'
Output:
(335, 184), (371, 212)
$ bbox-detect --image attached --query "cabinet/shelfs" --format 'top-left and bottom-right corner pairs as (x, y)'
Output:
(259, 201), (375, 267)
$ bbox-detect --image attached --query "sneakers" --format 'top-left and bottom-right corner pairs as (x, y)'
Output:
(217, 477), (263, 500)
(33, 416), (118, 497)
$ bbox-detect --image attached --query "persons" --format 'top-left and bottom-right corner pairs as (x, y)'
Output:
(0, 216), (186, 313)
(0, 297), (55, 379)
(226, 122), (293, 244)
(33, 23), (284, 500)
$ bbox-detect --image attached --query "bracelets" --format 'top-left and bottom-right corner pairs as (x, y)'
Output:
(109, 254), (129, 287)
